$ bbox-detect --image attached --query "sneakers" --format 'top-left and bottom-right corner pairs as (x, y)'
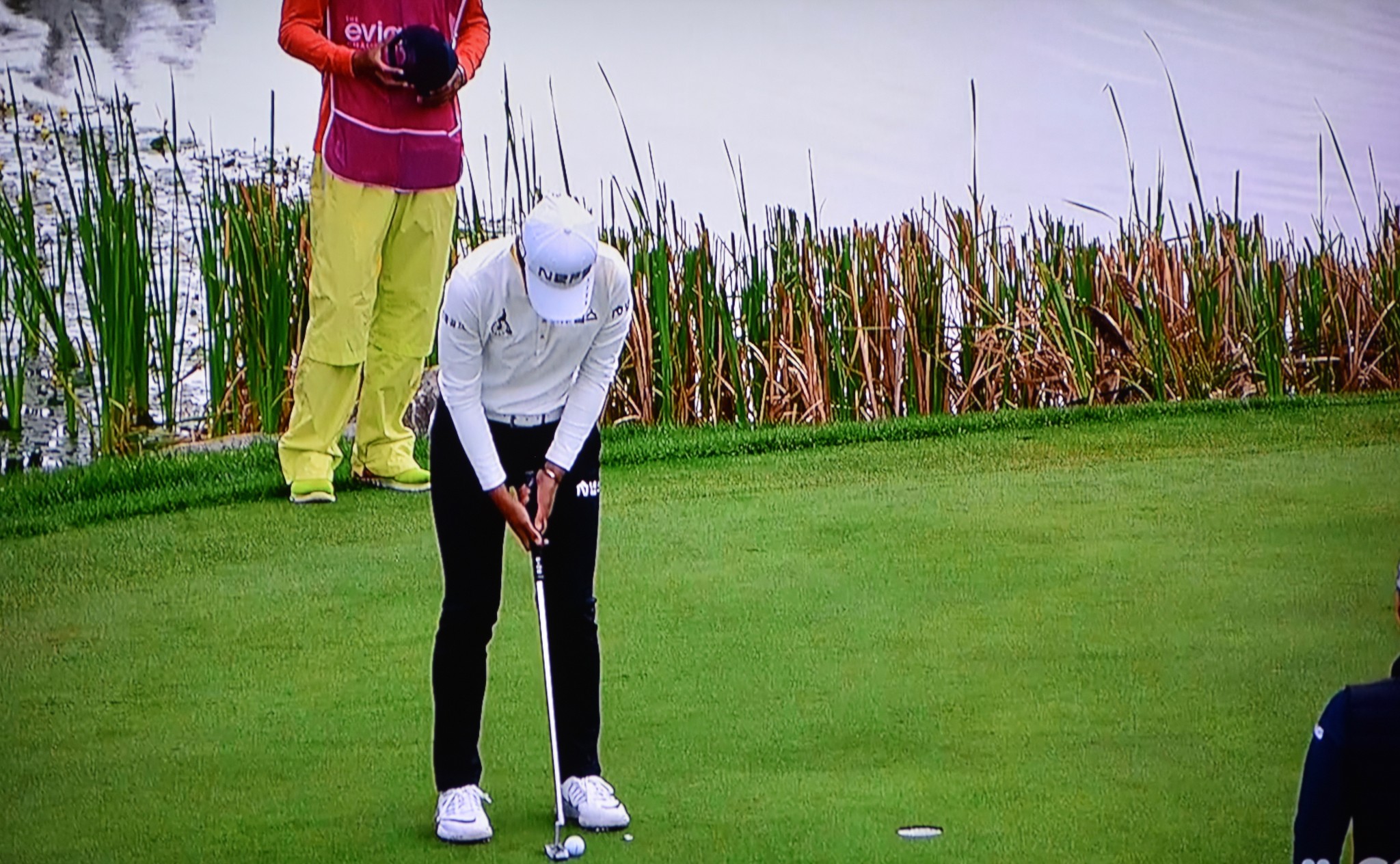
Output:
(554, 777), (631, 827)
(437, 784), (493, 842)
(289, 479), (336, 502)
(355, 469), (432, 491)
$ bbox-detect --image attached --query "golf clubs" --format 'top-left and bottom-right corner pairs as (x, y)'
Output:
(524, 468), (569, 862)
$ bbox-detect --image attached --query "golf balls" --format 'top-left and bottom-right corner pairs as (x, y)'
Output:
(564, 835), (585, 857)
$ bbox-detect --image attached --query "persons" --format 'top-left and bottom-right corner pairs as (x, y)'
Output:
(428, 190), (636, 845)
(278, 0), (491, 505)
(1291, 568), (1400, 864)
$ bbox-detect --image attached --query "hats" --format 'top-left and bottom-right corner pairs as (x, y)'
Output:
(384, 25), (458, 95)
(520, 192), (598, 320)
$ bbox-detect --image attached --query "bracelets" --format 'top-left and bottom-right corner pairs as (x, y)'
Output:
(540, 464), (562, 485)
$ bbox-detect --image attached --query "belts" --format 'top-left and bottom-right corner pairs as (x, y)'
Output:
(485, 408), (563, 427)
